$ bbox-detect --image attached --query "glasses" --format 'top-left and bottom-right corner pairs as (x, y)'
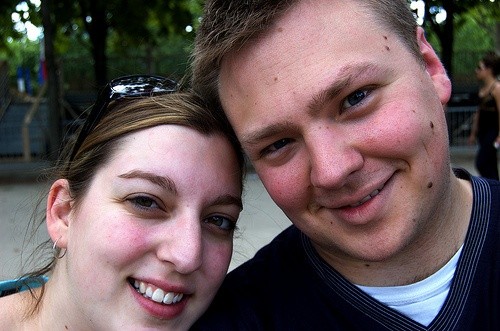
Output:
(477, 65), (485, 70)
(66, 74), (191, 178)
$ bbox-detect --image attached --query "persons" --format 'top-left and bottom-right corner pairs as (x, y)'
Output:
(186, 0), (500, 331)
(0, 83), (247, 331)
(468, 51), (500, 181)
